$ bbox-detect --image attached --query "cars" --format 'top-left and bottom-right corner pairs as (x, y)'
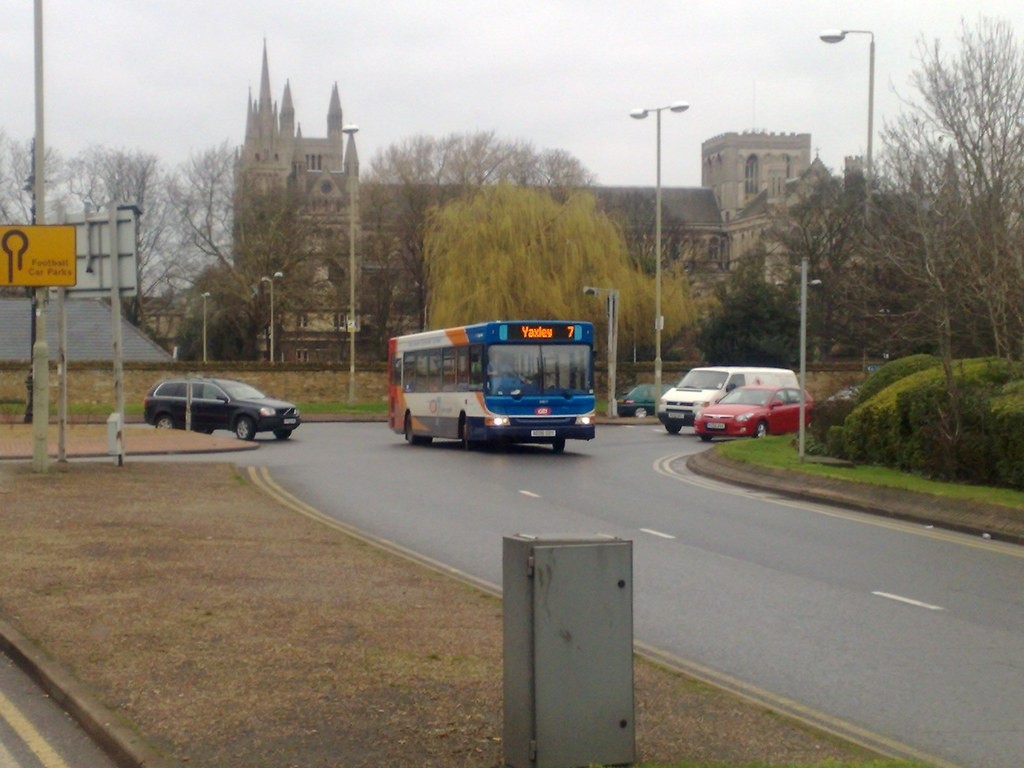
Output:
(826, 385), (864, 402)
(694, 385), (815, 440)
(614, 383), (675, 419)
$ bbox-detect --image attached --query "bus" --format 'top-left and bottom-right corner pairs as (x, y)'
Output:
(389, 319), (597, 455)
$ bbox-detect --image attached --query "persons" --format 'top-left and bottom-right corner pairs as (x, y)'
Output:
(487, 352), (513, 376)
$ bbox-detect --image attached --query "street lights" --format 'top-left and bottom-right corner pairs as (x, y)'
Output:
(342, 125), (363, 398)
(797, 279), (824, 457)
(583, 286), (615, 419)
(630, 104), (691, 411)
(202, 292), (213, 361)
(260, 271), (283, 361)
(110, 199), (145, 465)
(821, 29), (876, 282)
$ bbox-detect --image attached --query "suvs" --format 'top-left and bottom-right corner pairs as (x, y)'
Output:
(146, 378), (301, 439)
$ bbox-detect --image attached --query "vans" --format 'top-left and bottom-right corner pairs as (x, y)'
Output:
(657, 364), (800, 434)
